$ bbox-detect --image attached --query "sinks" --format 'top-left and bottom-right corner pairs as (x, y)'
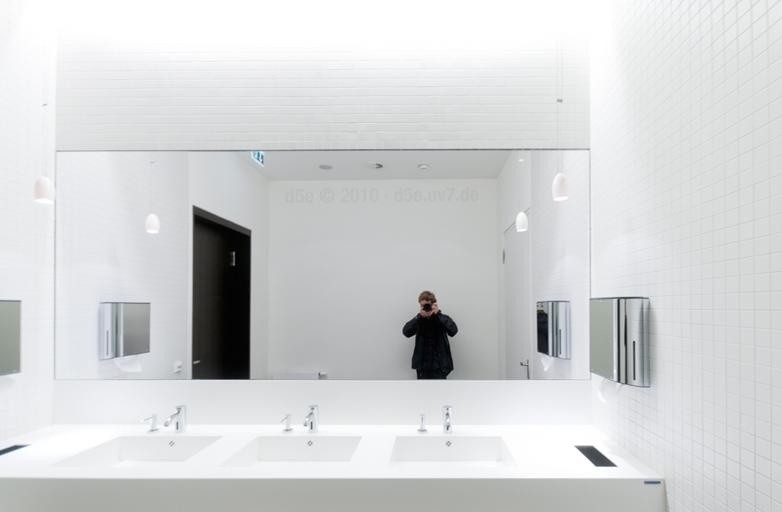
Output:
(62, 431), (223, 466)
(239, 432), (365, 464)
(391, 430), (510, 464)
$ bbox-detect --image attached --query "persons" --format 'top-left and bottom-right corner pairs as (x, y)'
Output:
(402, 290), (458, 379)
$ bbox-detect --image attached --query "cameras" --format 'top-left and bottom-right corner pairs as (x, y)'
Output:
(422, 303), (432, 313)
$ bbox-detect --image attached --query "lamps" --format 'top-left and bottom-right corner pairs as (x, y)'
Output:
(368, 162), (382, 169)
(146, 156), (160, 236)
(419, 164), (429, 172)
(551, 98), (570, 203)
(32, 103), (56, 207)
(515, 157), (529, 234)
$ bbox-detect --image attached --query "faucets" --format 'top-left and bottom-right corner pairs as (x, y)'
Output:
(442, 403), (455, 433)
(162, 403), (188, 431)
(519, 358), (530, 380)
(301, 403), (320, 432)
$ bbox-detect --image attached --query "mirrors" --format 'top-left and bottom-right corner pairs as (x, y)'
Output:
(53, 148), (594, 379)
